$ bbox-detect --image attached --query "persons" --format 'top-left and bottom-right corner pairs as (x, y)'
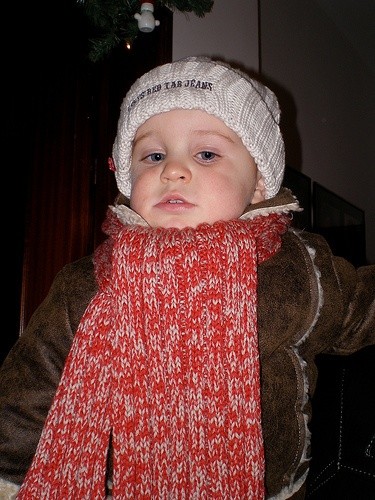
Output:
(0, 57), (375, 500)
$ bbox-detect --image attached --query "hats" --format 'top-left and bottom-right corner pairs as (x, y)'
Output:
(108, 56), (285, 199)
